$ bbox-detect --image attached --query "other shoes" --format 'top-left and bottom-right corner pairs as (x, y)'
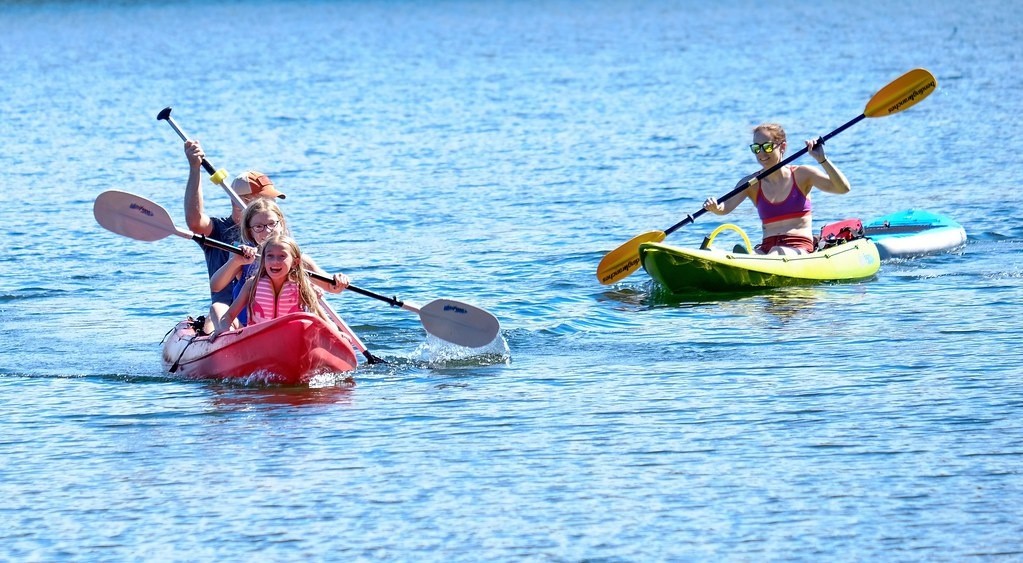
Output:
(733, 244), (748, 254)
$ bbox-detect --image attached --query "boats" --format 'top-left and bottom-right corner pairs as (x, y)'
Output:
(637, 237), (882, 294)
(161, 311), (358, 383)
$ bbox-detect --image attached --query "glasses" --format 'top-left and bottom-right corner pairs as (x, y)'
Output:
(250, 219), (281, 233)
(749, 141), (783, 154)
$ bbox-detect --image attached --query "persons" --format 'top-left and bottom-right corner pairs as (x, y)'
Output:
(184, 138), (352, 344)
(702, 124), (851, 255)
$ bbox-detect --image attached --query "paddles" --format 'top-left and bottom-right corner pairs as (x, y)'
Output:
(593, 68), (938, 285)
(90, 104), (499, 367)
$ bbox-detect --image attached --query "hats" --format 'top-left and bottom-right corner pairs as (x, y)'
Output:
(230, 171), (286, 199)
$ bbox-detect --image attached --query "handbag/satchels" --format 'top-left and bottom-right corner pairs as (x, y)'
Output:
(819, 218), (865, 244)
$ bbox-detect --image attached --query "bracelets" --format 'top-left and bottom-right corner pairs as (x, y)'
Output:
(818, 158), (827, 164)
